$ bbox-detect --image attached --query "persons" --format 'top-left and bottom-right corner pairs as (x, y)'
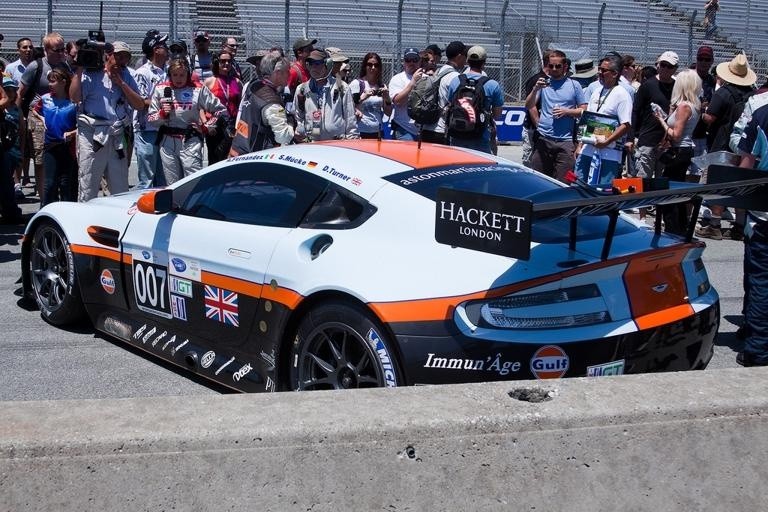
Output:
(522, 46), (768, 238)
(701, 1), (718, 35)
(734, 102), (768, 368)
(1, 30), (505, 238)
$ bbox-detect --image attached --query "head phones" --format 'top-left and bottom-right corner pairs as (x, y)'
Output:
(212, 52), (220, 66)
(164, 56), (193, 78)
(301, 49), (333, 71)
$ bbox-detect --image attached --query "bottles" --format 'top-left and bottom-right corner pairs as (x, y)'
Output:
(650, 103), (668, 120)
(593, 127), (613, 140)
(553, 104), (560, 119)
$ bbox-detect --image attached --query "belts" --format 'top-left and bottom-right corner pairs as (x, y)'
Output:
(165, 127), (190, 135)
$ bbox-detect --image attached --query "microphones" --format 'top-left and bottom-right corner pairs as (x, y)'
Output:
(315, 66), (335, 82)
(164, 86), (173, 116)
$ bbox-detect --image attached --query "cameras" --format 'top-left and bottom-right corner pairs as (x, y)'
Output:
(373, 89), (383, 97)
(542, 79), (552, 86)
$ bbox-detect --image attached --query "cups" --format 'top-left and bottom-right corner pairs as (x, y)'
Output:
(579, 124), (587, 135)
(542, 79), (550, 86)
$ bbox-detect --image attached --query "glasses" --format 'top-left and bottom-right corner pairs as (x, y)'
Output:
(227, 44), (239, 47)
(661, 63), (675, 69)
(195, 39), (208, 42)
(698, 57), (711, 62)
(549, 64), (562, 68)
(367, 63), (378, 67)
(219, 59), (231, 63)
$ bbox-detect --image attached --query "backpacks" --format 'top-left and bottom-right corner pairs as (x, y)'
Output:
(448, 75), (489, 141)
(407, 68), (454, 124)
(726, 85), (754, 139)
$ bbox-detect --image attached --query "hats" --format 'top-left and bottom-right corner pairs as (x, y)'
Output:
(293, 38), (349, 64)
(193, 31), (209, 40)
(142, 29), (186, 53)
(716, 54), (757, 86)
(571, 58), (598, 79)
(660, 51), (679, 65)
(697, 46), (712, 56)
(404, 40), (487, 61)
(246, 50), (270, 64)
(90, 31), (131, 54)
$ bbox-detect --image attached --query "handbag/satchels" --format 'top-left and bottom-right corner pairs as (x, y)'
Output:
(22, 91), (34, 116)
(587, 153), (601, 185)
(1, 107), (18, 149)
(655, 139), (680, 167)
(216, 125), (236, 154)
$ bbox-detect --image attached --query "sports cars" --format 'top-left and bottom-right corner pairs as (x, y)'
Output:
(15, 133), (767, 402)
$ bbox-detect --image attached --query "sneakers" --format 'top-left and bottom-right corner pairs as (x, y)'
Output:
(0, 215), (22, 224)
(695, 208), (745, 240)
(15, 185), (24, 199)
(736, 326), (754, 366)
(23, 177), (35, 187)
